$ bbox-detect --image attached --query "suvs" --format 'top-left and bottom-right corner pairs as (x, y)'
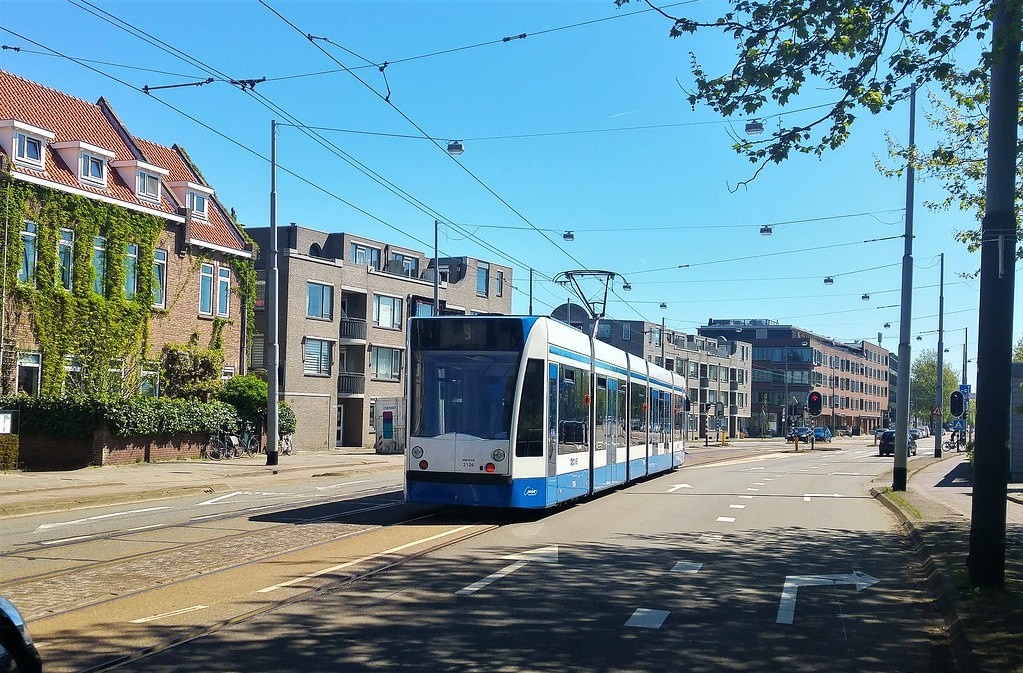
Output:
(877, 429), (917, 457)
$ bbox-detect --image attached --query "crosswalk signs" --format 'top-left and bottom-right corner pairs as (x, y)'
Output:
(953, 420), (966, 430)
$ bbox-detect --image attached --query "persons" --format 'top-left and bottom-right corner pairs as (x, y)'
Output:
(950, 430), (959, 443)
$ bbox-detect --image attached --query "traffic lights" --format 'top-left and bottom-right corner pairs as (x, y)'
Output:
(793, 404), (798, 416)
(788, 404), (793, 416)
(704, 403), (711, 412)
(701, 403), (704, 412)
(807, 391), (823, 418)
(950, 390), (964, 418)
(888, 410), (894, 419)
(717, 410), (722, 417)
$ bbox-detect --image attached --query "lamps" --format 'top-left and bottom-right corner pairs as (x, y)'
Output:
(745, 117), (764, 136)
(916, 336), (922, 342)
(944, 348), (949, 353)
(884, 322), (890, 329)
(447, 140), (464, 155)
(760, 225), (773, 237)
(862, 293), (870, 301)
(660, 302), (667, 309)
(623, 283), (632, 291)
(563, 231), (575, 241)
(824, 276), (834, 285)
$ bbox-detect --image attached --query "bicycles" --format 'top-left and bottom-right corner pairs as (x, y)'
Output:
(277, 427), (294, 457)
(942, 434), (968, 452)
(206, 420), (260, 460)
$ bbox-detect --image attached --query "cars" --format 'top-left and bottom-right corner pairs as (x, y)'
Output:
(811, 426), (832, 443)
(941, 425), (956, 436)
(875, 428), (890, 440)
(740, 427), (750, 438)
(908, 426), (936, 440)
(786, 426), (815, 443)
(600, 413), (671, 435)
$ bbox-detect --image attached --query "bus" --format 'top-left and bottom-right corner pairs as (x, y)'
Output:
(403, 270), (687, 511)
